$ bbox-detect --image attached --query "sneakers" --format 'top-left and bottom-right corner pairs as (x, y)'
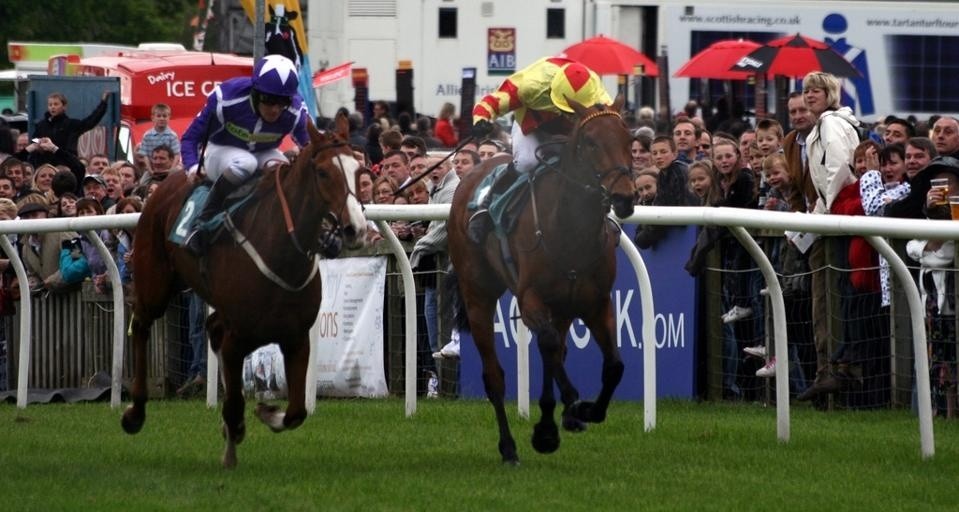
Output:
(744, 345), (767, 358)
(432, 352), (445, 361)
(724, 308), (751, 323)
(441, 341), (460, 360)
(757, 357), (779, 377)
(797, 378), (840, 400)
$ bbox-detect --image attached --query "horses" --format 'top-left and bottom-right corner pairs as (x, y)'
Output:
(121, 110), (367, 468)
(447, 83), (636, 466)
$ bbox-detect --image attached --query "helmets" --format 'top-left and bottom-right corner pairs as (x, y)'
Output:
(254, 55), (300, 97)
(549, 62), (599, 113)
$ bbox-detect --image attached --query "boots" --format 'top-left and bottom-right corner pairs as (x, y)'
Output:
(468, 161), (521, 245)
(187, 176), (236, 259)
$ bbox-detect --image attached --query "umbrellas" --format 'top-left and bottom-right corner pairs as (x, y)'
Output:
(557, 34), (661, 82)
(675, 38), (764, 82)
(731, 32), (863, 82)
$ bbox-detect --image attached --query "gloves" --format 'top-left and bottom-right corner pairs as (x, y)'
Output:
(188, 161), (205, 184)
(472, 120), (494, 144)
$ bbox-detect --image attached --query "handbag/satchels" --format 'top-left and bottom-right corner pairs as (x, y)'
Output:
(814, 113), (866, 223)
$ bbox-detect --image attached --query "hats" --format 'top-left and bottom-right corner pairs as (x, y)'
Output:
(53, 172), (76, 197)
(18, 203), (46, 215)
(82, 176), (104, 185)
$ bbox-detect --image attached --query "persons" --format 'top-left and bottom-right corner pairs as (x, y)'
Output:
(181, 55), (359, 259)
(688, 161), (715, 200)
(784, 93), (817, 211)
(374, 101), (390, 120)
(2, 199), (18, 267)
(740, 130), (756, 160)
(12, 195), (90, 295)
(636, 106), (657, 127)
(31, 90), (112, 159)
(16, 131), (30, 155)
(635, 173), (660, 202)
(758, 152), (787, 298)
(139, 103), (181, 176)
(478, 133), (502, 163)
(673, 118), (703, 160)
(931, 116), (959, 155)
(830, 143), (880, 290)
(649, 134), (690, 208)
(420, 116), (439, 148)
(631, 135), (653, 174)
(0, 177), (18, 202)
(892, 156), (957, 313)
(374, 177), (414, 247)
(51, 170), (82, 195)
(115, 199), (146, 282)
(397, 112), (417, 139)
(455, 148), (480, 179)
(410, 157), (460, 358)
(465, 56), (609, 245)
(121, 164), (139, 195)
(380, 130), (402, 156)
(750, 150), (767, 170)
(755, 313), (788, 378)
(102, 168), (123, 195)
(885, 155), (959, 413)
(88, 152), (110, 173)
(677, 101), (705, 127)
(75, 196), (115, 296)
(743, 118), (782, 356)
(355, 168), (377, 254)
(406, 175), (439, 401)
(802, 72), (861, 395)
(697, 128), (712, 155)
(435, 103), (460, 146)
(34, 164), (55, 198)
(58, 192), (80, 218)
(22, 164), (33, 190)
(2, 157), (23, 190)
(383, 150), (410, 182)
(883, 114), (915, 147)
(861, 136), (937, 306)
(706, 138), (756, 324)
(143, 146), (179, 198)
